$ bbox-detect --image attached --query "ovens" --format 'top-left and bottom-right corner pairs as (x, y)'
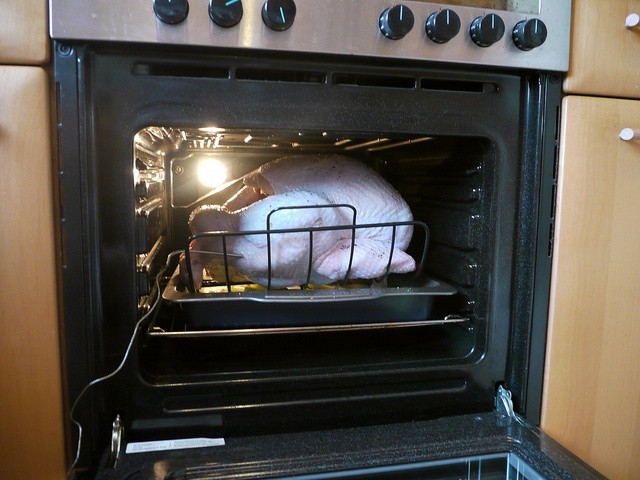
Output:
(47, 0), (609, 480)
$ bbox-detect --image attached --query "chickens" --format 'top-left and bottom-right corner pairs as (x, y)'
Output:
(174, 152), (416, 291)
